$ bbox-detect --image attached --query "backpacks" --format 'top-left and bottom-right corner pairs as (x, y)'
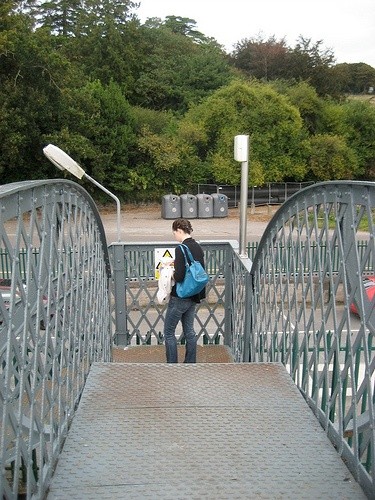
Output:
(176, 243), (208, 298)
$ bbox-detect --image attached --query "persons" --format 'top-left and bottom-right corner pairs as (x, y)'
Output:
(164, 218), (206, 363)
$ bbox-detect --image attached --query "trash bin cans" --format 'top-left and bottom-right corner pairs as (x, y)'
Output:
(161, 193), (228, 219)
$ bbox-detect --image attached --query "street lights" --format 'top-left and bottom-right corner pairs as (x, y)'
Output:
(43, 143), (121, 242)
(233, 135), (253, 273)
